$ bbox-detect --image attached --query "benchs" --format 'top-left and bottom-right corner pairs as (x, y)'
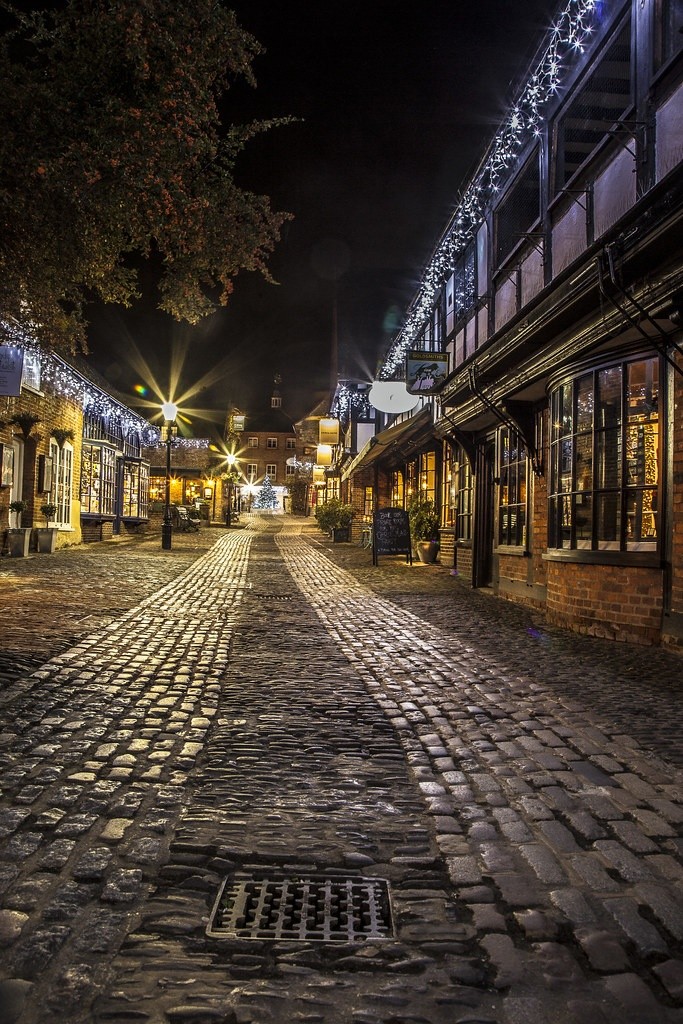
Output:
(175, 506), (200, 531)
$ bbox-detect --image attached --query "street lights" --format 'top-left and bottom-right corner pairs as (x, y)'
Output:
(162, 401), (177, 550)
(225, 454), (235, 526)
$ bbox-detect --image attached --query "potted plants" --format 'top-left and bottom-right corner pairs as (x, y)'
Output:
(50, 426), (73, 450)
(7, 500), (31, 557)
(36, 503), (58, 554)
(12, 411), (42, 439)
(315, 498), (360, 543)
(408, 489), (440, 564)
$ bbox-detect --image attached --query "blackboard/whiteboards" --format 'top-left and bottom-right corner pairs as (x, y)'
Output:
(372, 506), (410, 555)
